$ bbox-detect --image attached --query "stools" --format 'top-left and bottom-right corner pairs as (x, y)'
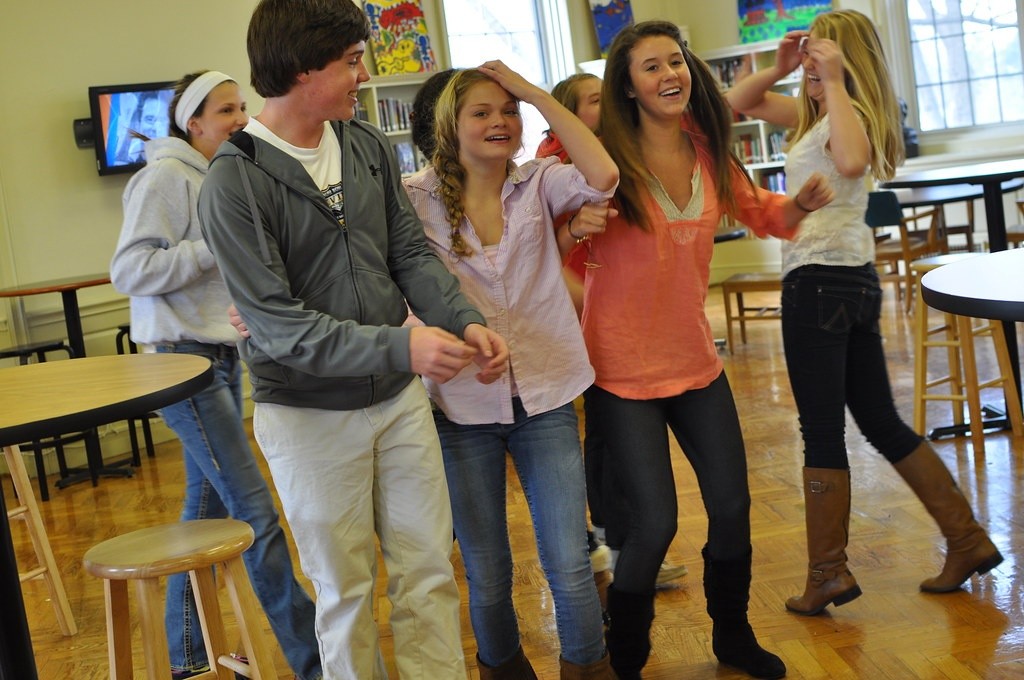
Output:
(85, 518), (279, 679)
(723, 272), (784, 352)
(115, 321), (156, 467)
(0, 340), (85, 500)
(909, 255), (1024, 451)
(0, 445), (77, 638)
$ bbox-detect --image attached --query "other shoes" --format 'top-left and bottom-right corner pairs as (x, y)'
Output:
(657, 559), (687, 584)
(170, 656), (251, 680)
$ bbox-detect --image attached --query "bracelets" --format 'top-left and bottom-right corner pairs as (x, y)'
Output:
(793, 195), (813, 212)
(568, 215), (593, 254)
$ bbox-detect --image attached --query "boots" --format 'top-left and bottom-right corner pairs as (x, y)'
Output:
(476, 644), (537, 680)
(702, 542), (787, 680)
(892, 435), (1004, 593)
(602, 583), (655, 680)
(785, 467), (862, 615)
(559, 651), (615, 680)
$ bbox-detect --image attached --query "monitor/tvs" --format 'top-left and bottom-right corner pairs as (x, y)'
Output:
(88, 80), (177, 176)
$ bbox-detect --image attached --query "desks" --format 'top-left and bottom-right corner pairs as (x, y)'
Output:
(881, 156), (1023, 257)
(922, 249), (1024, 438)
(1, 275), (137, 489)
(0, 357), (215, 680)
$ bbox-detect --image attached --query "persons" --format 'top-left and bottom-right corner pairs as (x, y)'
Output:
(390, 64), (624, 680)
(195, 0), (511, 680)
(725, 9), (1005, 612)
(541, 68), (687, 627)
(555, 20), (837, 680)
(110, 69), (320, 680)
(129, 90), (158, 161)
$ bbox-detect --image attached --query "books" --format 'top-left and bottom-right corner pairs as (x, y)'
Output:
(354, 97), (430, 175)
(712, 58), (807, 227)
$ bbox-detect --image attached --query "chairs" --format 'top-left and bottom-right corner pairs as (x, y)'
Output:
(864, 191), (975, 311)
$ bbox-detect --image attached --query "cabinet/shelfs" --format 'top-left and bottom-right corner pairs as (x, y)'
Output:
(350, 79), (430, 183)
(707, 40), (809, 242)
